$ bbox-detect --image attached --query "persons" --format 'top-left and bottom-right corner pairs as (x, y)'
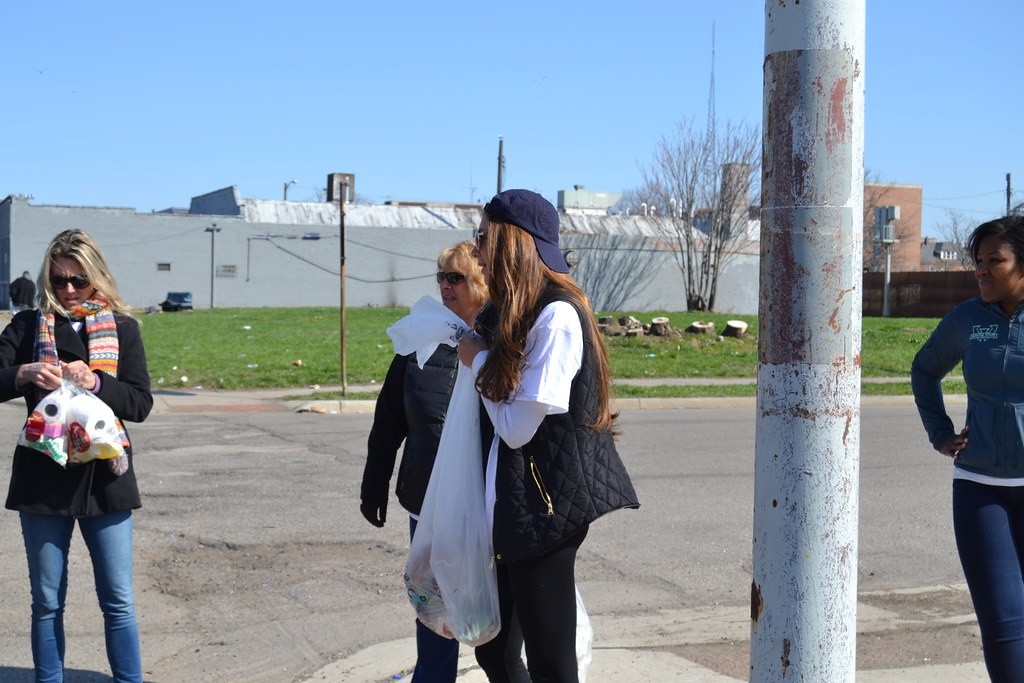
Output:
(456, 188), (641, 683)
(0, 229), (154, 683)
(360, 240), (491, 683)
(911, 216), (1024, 683)
(9, 270), (37, 318)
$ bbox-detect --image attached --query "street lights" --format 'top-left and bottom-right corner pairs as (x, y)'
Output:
(283, 180), (297, 201)
(205, 223), (222, 309)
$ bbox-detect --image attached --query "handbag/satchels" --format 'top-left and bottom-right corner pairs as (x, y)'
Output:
(386, 294), (501, 648)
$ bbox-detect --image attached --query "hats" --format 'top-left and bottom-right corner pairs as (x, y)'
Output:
(483, 189), (569, 274)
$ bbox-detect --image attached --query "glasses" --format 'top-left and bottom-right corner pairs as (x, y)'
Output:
(436, 272), (465, 285)
(50, 274), (90, 290)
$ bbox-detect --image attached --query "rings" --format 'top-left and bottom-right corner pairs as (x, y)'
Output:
(77, 373), (80, 378)
